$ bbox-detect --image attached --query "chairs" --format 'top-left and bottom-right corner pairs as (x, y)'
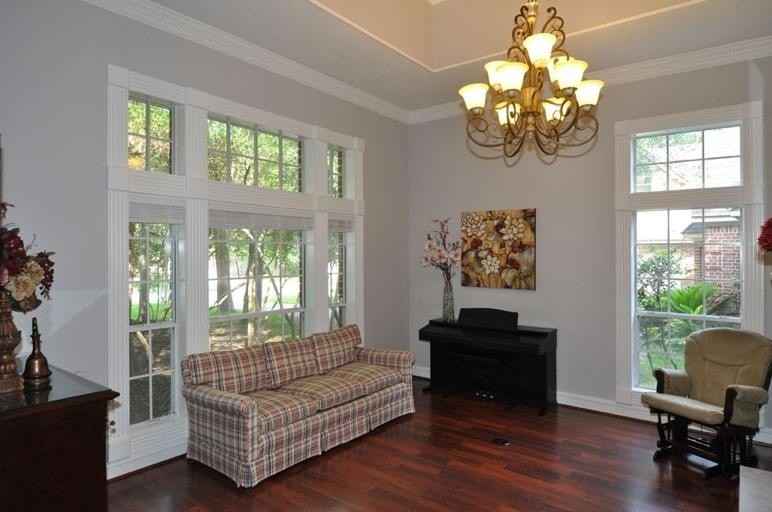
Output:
(640, 325), (771, 481)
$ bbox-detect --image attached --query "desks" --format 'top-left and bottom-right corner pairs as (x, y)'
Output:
(0, 356), (120, 511)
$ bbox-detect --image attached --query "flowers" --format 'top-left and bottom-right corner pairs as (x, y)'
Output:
(0, 200), (55, 314)
(420, 215), (463, 284)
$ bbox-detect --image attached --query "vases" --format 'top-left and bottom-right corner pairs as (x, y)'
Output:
(0, 290), (23, 394)
(442, 285), (454, 321)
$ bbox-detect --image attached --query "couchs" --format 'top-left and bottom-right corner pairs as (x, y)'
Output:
(179, 323), (417, 486)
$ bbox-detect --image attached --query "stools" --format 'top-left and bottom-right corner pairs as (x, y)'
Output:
(442, 346), (521, 409)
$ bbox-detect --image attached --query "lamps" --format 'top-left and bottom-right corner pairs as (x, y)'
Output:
(459, 0), (602, 160)
